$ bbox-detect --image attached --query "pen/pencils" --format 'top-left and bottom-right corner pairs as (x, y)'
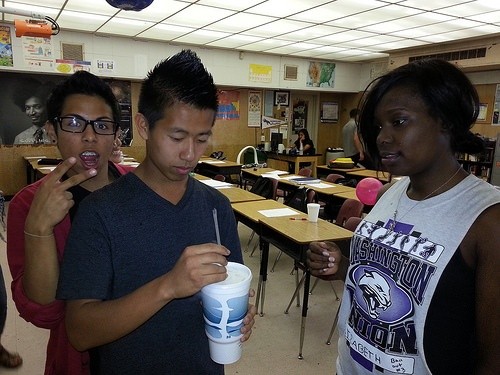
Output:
(290, 218), (307, 221)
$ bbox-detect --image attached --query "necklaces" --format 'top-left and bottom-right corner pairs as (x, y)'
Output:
(387, 165), (462, 234)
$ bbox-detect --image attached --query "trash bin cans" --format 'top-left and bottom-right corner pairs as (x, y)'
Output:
(327, 148), (344, 164)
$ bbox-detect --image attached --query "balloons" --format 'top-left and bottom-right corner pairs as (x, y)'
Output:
(356, 178), (384, 205)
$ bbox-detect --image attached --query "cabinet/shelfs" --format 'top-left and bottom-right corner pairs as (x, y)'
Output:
(453, 136), (497, 183)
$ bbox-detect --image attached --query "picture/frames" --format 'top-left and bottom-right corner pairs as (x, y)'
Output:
(274, 90), (290, 106)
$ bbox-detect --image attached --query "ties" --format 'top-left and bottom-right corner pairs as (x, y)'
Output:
(34, 129), (43, 144)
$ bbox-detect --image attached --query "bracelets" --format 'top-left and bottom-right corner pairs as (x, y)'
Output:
(24, 231), (54, 238)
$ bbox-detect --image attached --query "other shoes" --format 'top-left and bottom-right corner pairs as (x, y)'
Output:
(0, 343), (22, 367)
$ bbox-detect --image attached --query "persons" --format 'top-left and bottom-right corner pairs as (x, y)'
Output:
(306, 61), (500, 375)
(11, 84), (55, 145)
(342, 108), (372, 169)
(7, 70), (136, 375)
(293, 129), (314, 172)
(55, 47), (256, 375)
(0, 263), (23, 368)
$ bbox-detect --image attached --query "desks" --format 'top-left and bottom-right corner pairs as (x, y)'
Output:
(22, 152), (392, 360)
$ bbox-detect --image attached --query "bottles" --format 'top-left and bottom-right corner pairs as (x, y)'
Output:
(327, 143), (343, 150)
(264, 141), (269, 151)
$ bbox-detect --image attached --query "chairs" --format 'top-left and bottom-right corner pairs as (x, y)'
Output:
(298, 168), (312, 176)
(326, 174), (344, 183)
(294, 198), (364, 307)
(214, 175), (225, 182)
(282, 218), (364, 346)
(255, 177), (278, 199)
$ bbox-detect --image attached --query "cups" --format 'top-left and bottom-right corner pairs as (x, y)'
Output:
(202, 261), (253, 364)
(307, 203), (321, 222)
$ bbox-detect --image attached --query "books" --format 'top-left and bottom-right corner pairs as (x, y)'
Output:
(455, 153), (490, 181)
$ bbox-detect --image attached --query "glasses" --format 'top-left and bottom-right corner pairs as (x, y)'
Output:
(52, 116), (120, 135)
